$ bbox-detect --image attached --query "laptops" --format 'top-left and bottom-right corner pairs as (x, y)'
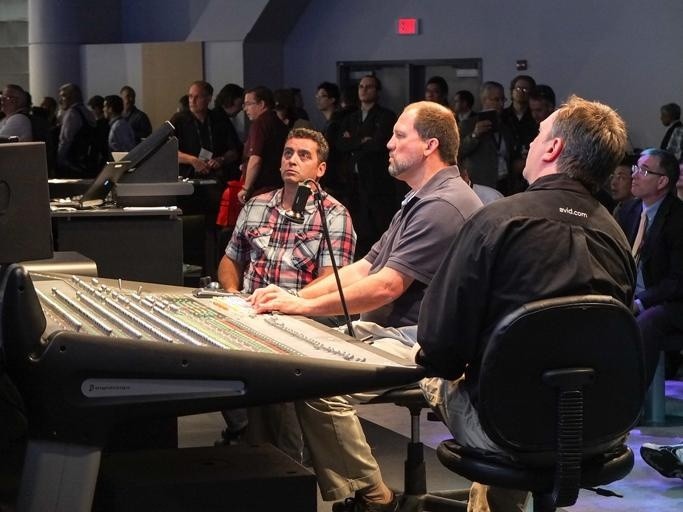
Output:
(52, 161), (132, 209)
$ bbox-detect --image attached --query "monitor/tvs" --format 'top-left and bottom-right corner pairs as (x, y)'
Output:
(120, 120), (178, 182)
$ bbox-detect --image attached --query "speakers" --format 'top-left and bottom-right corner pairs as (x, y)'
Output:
(0, 141), (53, 265)
(103, 442), (317, 512)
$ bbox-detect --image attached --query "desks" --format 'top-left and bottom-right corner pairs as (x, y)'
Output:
(50, 197), (184, 290)
(45, 178), (224, 285)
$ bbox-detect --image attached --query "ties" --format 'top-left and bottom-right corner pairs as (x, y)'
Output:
(631, 212), (647, 258)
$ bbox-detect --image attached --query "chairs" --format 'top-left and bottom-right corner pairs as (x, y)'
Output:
(333, 381), (471, 512)
(433, 287), (651, 512)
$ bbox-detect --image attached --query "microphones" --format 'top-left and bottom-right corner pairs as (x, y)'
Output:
(285, 183), (312, 224)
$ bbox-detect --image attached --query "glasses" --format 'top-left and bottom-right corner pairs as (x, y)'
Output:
(631, 163), (663, 178)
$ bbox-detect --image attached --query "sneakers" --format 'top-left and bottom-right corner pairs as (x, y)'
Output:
(332, 491), (399, 511)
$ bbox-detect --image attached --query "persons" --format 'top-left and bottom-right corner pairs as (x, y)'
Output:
(214, 127), (356, 464)
(410, 91), (639, 512)
(457, 153), (504, 206)
(1, 71), (682, 276)
(613, 147), (683, 426)
(244, 101), (484, 510)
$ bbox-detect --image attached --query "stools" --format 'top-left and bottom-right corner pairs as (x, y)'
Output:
(630, 326), (681, 439)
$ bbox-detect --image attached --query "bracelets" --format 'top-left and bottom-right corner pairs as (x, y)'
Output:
(288, 286), (300, 298)
